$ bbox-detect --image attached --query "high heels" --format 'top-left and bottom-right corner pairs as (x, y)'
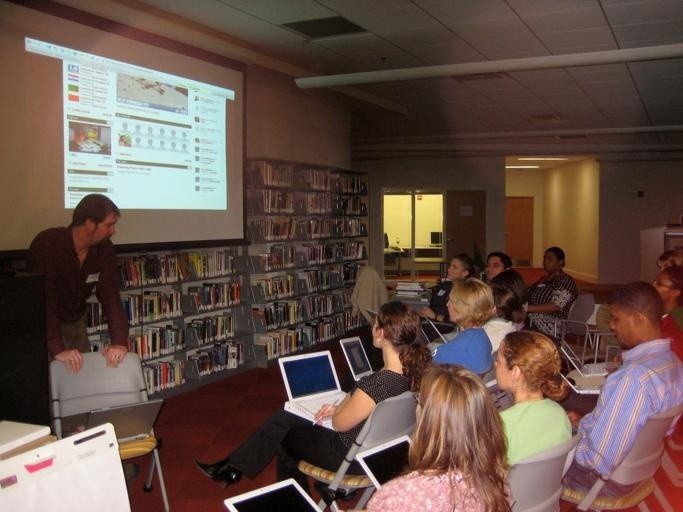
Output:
(192, 456), (244, 490)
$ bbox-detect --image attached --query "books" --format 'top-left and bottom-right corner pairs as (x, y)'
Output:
(77, 245), (246, 395)
(394, 280), (428, 299)
(249, 155), (373, 362)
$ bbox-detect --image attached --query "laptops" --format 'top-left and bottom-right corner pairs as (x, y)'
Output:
(278, 350), (348, 419)
(83, 398), (164, 440)
(355, 434), (417, 488)
(224, 478), (323, 512)
(339, 336), (373, 381)
(557, 369), (602, 396)
(559, 339), (607, 376)
(424, 314), (449, 344)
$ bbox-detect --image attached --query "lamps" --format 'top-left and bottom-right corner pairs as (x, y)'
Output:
(293, 41), (682, 90)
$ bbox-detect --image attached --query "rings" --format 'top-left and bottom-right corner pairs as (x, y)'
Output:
(114, 354), (119, 359)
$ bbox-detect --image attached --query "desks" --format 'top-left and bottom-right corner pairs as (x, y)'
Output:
(399, 245), (444, 278)
(382, 246), (405, 278)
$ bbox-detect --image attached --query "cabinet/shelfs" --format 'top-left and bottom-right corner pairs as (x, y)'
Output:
(234, 154), (371, 375)
(84, 244), (259, 405)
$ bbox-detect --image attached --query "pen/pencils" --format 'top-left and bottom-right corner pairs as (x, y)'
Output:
(313, 399), (339, 426)
(74, 431), (105, 445)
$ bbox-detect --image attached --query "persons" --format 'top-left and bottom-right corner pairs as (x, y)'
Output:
(24, 194), (129, 376)
(493, 329), (574, 467)
(556, 278), (683, 502)
(341, 362), (513, 512)
(428, 275), (495, 379)
(192, 300), (432, 500)
(415, 241), (683, 370)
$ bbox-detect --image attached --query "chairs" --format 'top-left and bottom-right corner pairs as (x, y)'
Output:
(41, 343), (173, 512)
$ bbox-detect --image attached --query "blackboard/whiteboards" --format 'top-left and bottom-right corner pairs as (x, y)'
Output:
(0, 423), (132, 512)
